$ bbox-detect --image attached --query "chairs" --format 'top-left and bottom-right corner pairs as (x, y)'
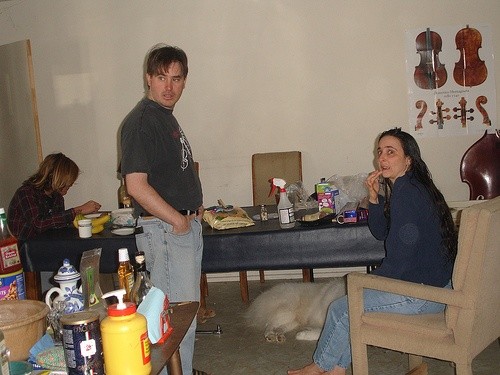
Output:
(345, 196), (500, 375)
(252, 151), (312, 283)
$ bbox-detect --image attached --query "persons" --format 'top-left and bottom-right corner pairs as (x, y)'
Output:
(8, 153), (101, 248)
(120, 45), (206, 375)
(288, 127), (458, 375)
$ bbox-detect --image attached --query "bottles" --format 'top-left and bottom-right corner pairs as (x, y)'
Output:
(0, 208), (21, 274)
(260, 204), (268, 221)
(117, 176), (132, 208)
(118, 248), (134, 303)
(131, 251), (150, 309)
(0, 329), (11, 375)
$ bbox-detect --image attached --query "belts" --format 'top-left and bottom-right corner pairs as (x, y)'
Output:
(139, 209), (199, 218)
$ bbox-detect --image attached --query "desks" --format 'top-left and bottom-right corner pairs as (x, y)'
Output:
(19, 206), (386, 310)
(20, 210), (145, 301)
(150, 301), (201, 375)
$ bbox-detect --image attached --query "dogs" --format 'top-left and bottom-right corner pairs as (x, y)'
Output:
(245, 274), (349, 343)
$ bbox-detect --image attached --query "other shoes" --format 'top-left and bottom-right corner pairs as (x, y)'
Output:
(192, 368), (207, 375)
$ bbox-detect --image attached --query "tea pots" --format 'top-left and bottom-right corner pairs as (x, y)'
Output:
(45, 259), (85, 322)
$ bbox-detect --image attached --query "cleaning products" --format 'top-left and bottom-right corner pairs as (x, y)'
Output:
(266, 176), (297, 229)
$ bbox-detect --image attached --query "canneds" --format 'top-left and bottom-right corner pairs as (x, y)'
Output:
(59, 311), (106, 375)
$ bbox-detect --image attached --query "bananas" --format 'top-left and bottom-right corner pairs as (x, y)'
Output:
(73, 210), (110, 234)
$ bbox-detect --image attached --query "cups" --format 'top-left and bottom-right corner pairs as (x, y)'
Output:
(60, 311), (107, 375)
(77, 219), (93, 238)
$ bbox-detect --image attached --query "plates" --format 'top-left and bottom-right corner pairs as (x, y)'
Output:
(111, 228), (135, 235)
(295, 212), (336, 226)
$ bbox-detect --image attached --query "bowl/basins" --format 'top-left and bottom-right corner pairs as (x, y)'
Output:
(112, 208), (135, 226)
(0, 300), (49, 361)
